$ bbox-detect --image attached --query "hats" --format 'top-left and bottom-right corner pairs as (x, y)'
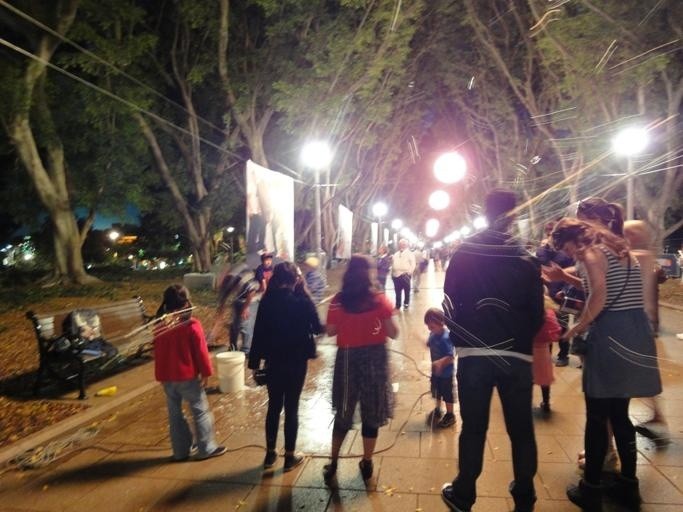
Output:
(260, 252), (273, 260)
(303, 257), (320, 270)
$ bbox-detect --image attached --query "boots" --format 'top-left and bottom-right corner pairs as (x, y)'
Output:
(605, 472), (643, 512)
(566, 478), (605, 511)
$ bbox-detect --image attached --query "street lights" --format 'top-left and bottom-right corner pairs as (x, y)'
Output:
(392, 216), (490, 258)
(611, 123), (650, 222)
(371, 200), (387, 252)
(299, 130), (336, 290)
(108, 230), (119, 263)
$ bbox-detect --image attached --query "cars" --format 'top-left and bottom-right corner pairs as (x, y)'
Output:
(119, 255), (191, 272)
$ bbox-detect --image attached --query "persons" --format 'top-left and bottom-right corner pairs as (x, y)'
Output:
(429, 240), (463, 271)
(540, 197), (665, 511)
(442, 191), (544, 511)
(376, 239), (428, 309)
(531, 285), (562, 416)
(152, 283), (226, 461)
(423, 307), (457, 427)
(234, 250), (327, 471)
(322, 254), (398, 477)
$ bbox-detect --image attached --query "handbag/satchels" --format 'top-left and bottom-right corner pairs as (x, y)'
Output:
(570, 316), (603, 360)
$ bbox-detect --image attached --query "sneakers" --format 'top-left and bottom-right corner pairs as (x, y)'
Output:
(439, 414), (456, 428)
(201, 446), (228, 458)
(540, 402), (551, 414)
(359, 460), (373, 479)
(262, 448), (279, 467)
(428, 410), (442, 420)
(441, 482), (472, 512)
(555, 357), (568, 367)
(322, 461), (338, 474)
(284, 452), (306, 470)
(174, 443), (198, 457)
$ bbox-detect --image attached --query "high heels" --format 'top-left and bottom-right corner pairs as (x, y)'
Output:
(578, 449), (622, 472)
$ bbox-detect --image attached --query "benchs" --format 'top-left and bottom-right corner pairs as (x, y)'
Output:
(23, 292), (159, 400)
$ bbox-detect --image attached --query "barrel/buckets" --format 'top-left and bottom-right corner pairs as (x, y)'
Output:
(215, 350), (247, 394)
(232, 297), (259, 350)
